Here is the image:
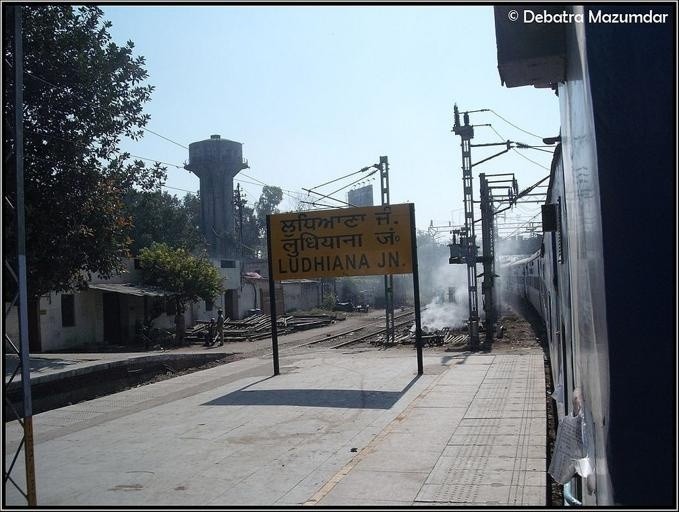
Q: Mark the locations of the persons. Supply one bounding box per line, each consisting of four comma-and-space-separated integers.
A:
214, 309, 224, 347
174, 308, 186, 348
203, 318, 217, 346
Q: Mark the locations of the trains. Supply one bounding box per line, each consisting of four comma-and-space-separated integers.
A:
494, 2, 677, 509
